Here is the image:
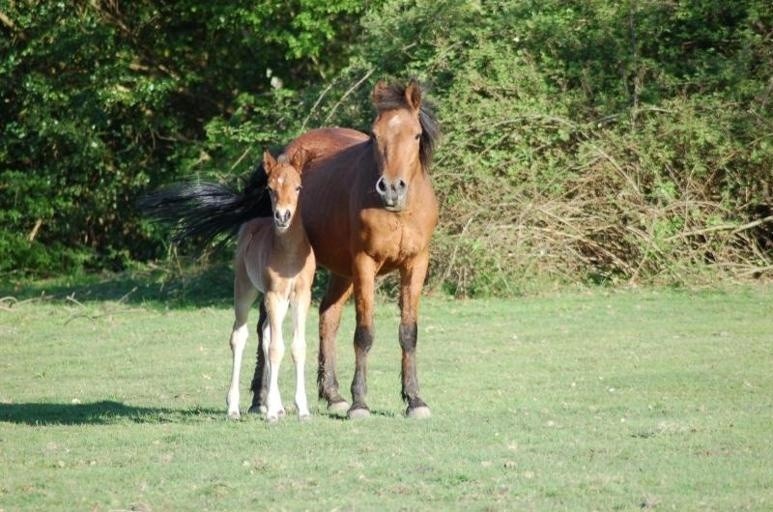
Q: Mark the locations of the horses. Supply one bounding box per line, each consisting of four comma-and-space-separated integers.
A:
225, 147, 316, 422
139, 76, 446, 422
